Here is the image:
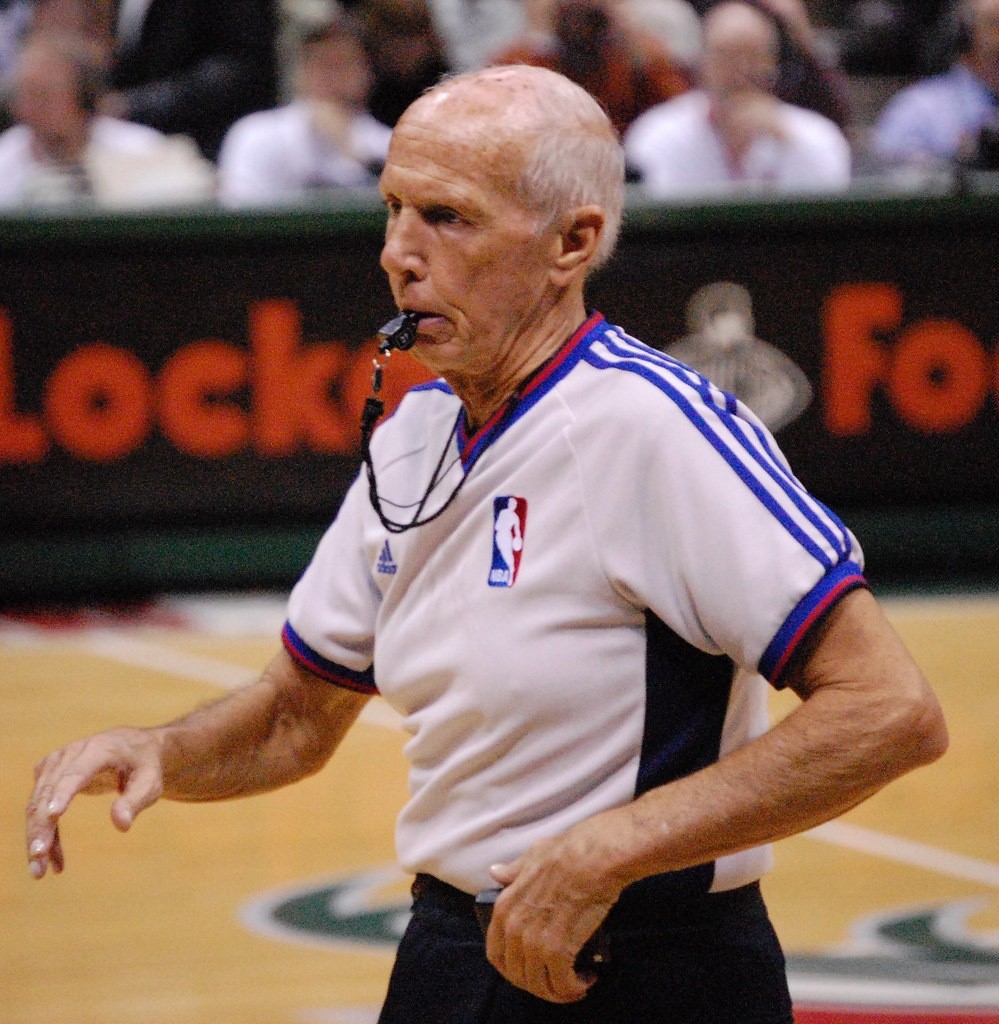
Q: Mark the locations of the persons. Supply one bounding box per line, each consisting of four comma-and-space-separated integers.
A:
0, 1, 999, 207
23, 62, 950, 1024
